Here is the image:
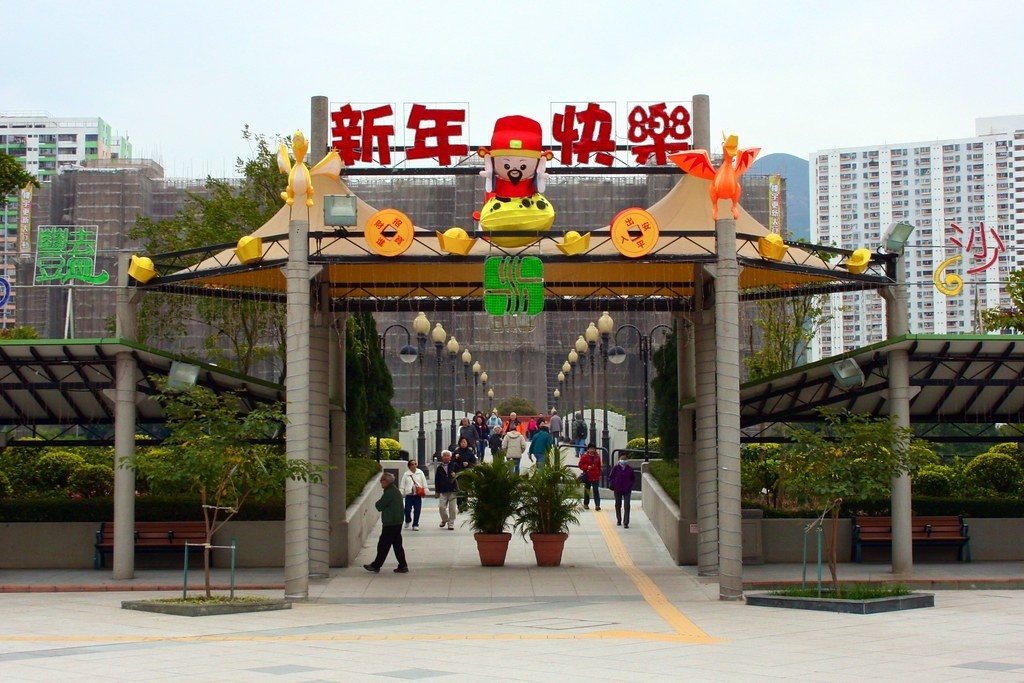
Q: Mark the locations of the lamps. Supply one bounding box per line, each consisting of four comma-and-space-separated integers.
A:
166, 361, 203, 393
879, 221, 917, 255
832, 358, 867, 387
322, 193, 362, 227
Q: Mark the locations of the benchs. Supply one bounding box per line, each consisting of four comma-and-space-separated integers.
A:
94, 521, 217, 569
848, 513, 974, 564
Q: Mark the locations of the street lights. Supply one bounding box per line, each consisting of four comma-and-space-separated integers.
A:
608, 323, 675, 461
413, 311, 430, 479
598, 311, 614, 466
373, 324, 418, 464
431, 322, 447, 463
568, 348, 578, 445
553, 389, 561, 412
585, 321, 600, 451
557, 371, 564, 442
574, 335, 588, 421
479, 371, 488, 415
561, 361, 571, 443
461, 348, 473, 418
446, 335, 460, 452
472, 360, 482, 415
487, 387, 496, 413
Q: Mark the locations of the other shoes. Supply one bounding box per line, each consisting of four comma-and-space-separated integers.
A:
439, 521, 446, 527
393, 568, 407, 573
595, 506, 600, 511
624, 524, 628, 528
412, 526, 419, 531
364, 564, 377, 571
584, 505, 589, 508
448, 525, 454, 530
617, 521, 621, 525
404, 521, 409, 528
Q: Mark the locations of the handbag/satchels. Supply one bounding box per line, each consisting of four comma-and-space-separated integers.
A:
416, 484, 425, 497
609, 467, 616, 490
451, 477, 459, 491
578, 473, 587, 483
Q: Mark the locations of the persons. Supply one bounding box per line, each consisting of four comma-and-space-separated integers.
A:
579, 442, 602, 511
607, 453, 635, 528
501, 424, 526, 475
529, 426, 553, 473
452, 437, 476, 512
478, 115, 553, 205
473, 411, 549, 446
363, 472, 409, 574
489, 426, 506, 465
460, 418, 479, 453
573, 413, 587, 458
434, 450, 464, 530
549, 411, 563, 447
400, 459, 429, 531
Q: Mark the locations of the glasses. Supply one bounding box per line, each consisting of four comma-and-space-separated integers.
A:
412, 464, 417, 465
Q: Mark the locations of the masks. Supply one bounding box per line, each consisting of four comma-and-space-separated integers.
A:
619, 459, 628, 464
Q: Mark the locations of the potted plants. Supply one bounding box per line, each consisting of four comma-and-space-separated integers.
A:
448, 447, 532, 567
512, 443, 592, 567
483, 396, 551, 424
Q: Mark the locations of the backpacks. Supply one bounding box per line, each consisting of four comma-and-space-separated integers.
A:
577, 420, 585, 438
